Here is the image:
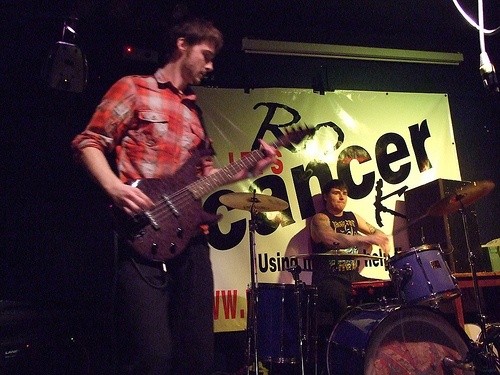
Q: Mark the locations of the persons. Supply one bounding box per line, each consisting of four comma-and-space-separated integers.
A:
69, 20, 277, 374
311, 179, 389, 315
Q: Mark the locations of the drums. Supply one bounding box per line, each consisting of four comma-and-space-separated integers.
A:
326, 302, 483, 375
245, 282, 319, 371
385, 243, 462, 309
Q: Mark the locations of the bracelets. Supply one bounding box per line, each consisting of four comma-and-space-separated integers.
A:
245, 166, 262, 177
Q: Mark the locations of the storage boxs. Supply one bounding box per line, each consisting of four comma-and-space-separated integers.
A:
405, 179, 483, 274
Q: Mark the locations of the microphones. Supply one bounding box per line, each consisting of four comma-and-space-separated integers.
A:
442, 356, 476, 371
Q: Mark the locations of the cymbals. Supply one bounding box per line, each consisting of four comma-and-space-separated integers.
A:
428, 181, 494, 216
290, 252, 386, 262
218, 192, 289, 212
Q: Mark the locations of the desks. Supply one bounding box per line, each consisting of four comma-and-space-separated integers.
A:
353, 272, 500, 329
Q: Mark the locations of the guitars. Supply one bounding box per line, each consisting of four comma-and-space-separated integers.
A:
112, 122, 318, 262
